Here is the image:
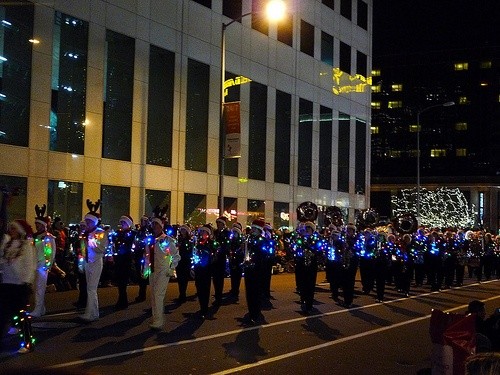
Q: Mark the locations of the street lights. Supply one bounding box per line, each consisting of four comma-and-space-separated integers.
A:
416, 100, 456, 215
216, 1, 288, 215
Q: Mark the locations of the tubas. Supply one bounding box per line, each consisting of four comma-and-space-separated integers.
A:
323, 206, 342, 226
296, 201, 319, 223
392, 212, 418, 234
358, 207, 380, 230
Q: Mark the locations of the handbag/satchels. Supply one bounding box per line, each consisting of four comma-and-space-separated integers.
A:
467, 300, 500, 355
429, 307, 477, 375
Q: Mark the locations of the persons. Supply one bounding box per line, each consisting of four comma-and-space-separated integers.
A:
0, 212, 500, 356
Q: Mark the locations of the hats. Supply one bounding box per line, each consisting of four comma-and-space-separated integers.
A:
232, 219, 273, 235
84, 199, 102, 225
142, 214, 148, 221
216, 216, 228, 226
200, 222, 212, 235
35, 204, 50, 232
151, 204, 169, 228
300, 221, 425, 244
180, 224, 191, 236
119, 215, 133, 228
9, 219, 33, 240
431, 228, 500, 241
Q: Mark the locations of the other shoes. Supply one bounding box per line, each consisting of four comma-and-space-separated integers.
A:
73, 302, 86, 308
149, 320, 164, 327
30, 304, 45, 317
292, 282, 464, 317
17, 346, 30, 353
78, 313, 99, 321
